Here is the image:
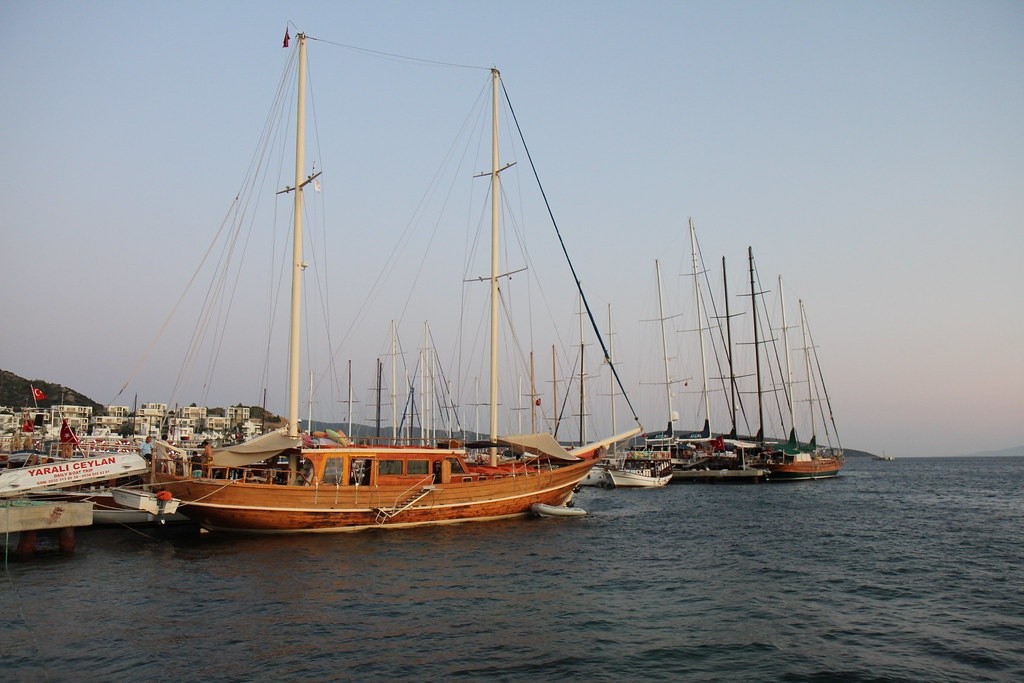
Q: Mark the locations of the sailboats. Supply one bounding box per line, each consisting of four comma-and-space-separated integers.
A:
0, 31, 845, 537
141, 33, 610, 536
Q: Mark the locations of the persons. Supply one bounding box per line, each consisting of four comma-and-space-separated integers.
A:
156, 434, 176, 474
188, 450, 203, 477
133, 436, 154, 461
201, 439, 214, 479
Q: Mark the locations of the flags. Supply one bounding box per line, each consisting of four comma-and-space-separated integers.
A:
21, 418, 34, 431
32, 386, 48, 399
60, 419, 79, 444
707, 436, 725, 451
641, 433, 648, 438
